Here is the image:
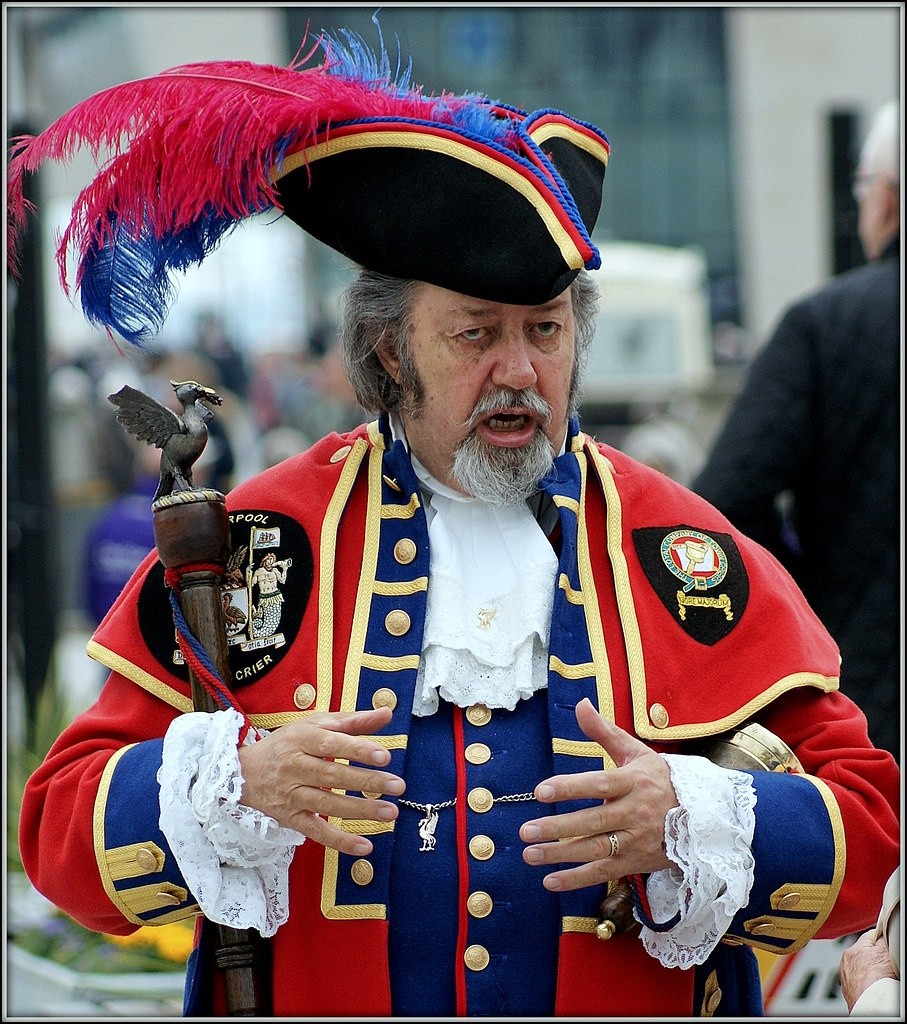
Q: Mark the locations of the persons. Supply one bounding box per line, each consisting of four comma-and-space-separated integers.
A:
37, 102, 903, 760
8, 13, 904, 1018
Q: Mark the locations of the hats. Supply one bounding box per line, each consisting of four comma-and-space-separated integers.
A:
7, 12, 610, 356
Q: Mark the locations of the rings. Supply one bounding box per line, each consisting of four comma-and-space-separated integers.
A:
605, 831, 619, 858
